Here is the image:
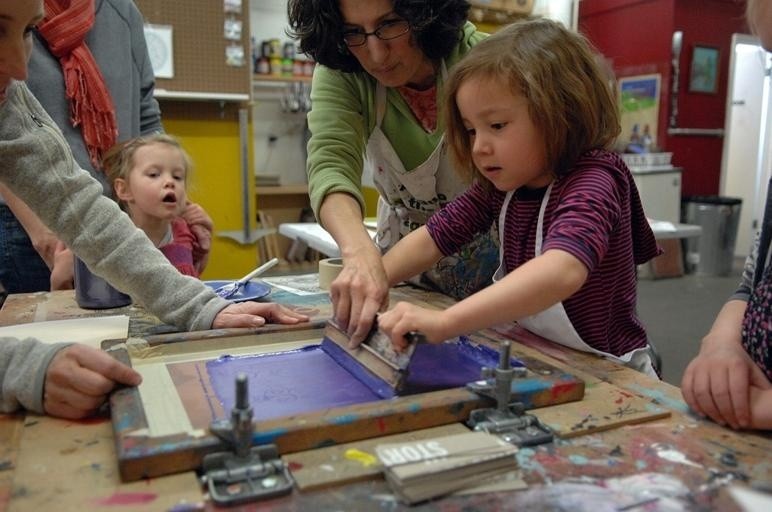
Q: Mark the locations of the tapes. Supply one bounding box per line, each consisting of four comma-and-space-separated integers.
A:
319, 257, 343, 292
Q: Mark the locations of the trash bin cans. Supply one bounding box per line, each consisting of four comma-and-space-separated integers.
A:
681, 194, 743, 276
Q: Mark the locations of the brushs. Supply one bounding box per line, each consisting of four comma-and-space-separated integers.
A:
216, 257, 279, 301
321, 304, 426, 399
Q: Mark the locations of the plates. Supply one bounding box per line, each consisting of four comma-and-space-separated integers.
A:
200, 281, 271, 303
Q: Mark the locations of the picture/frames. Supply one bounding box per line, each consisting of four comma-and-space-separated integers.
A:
615, 73, 662, 155
685, 41, 722, 97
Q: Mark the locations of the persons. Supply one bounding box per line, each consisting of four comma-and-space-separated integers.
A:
682, 2, 772, 434
378, 17, 663, 381
0, 0, 212, 292
0, 0, 310, 419
45, 132, 215, 291
286, 1, 497, 348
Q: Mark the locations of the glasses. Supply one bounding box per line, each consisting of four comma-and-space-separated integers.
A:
340, 16, 414, 46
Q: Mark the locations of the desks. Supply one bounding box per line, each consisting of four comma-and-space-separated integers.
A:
0, 274, 771, 512
